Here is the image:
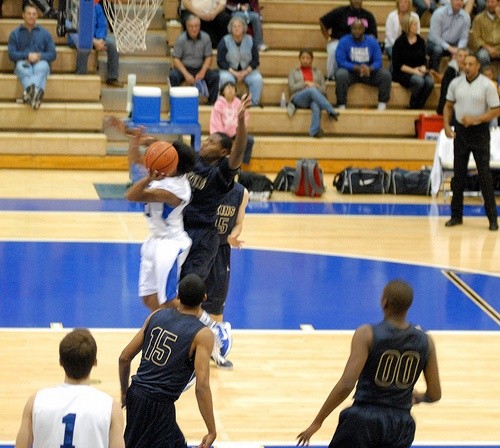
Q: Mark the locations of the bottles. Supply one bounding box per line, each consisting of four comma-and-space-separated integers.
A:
281, 92, 286, 108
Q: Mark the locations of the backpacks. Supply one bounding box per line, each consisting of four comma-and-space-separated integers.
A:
291, 159, 326, 198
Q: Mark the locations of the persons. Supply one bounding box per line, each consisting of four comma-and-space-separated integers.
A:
14, 328, 126, 448
334, 18, 392, 109
210, 81, 254, 164
8, 2, 56, 110
181, 0, 269, 51
169, 14, 220, 105
295, 279, 441, 448
119, 274, 216, 448
319, 0, 378, 80
443, 53, 500, 231
217, 16, 264, 109
32, 0, 57, 20
436, 48, 472, 126
481, 64, 500, 127
390, 14, 434, 109
412, 0, 500, 71
288, 48, 340, 138
384, 0, 420, 61
107, 92, 252, 392
68, 4, 124, 88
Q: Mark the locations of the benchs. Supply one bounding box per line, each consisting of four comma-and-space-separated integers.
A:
0, 0, 500, 175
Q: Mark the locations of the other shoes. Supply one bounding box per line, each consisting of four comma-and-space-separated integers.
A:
23, 87, 34, 103
332, 112, 340, 121
108, 78, 124, 88
56, 11, 66, 37
446, 218, 463, 226
489, 221, 498, 231
32, 91, 43, 109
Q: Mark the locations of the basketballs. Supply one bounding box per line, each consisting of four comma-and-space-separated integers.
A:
144, 140, 179, 177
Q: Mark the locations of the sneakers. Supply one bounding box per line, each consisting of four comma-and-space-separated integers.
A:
213, 321, 231, 357
211, 353, 233, 367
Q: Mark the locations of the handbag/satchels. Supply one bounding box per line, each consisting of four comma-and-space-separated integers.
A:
333, 167, 388, 194
388, 168, 431, 197
274, 167, 297, 191
239, 173, 274, 200
